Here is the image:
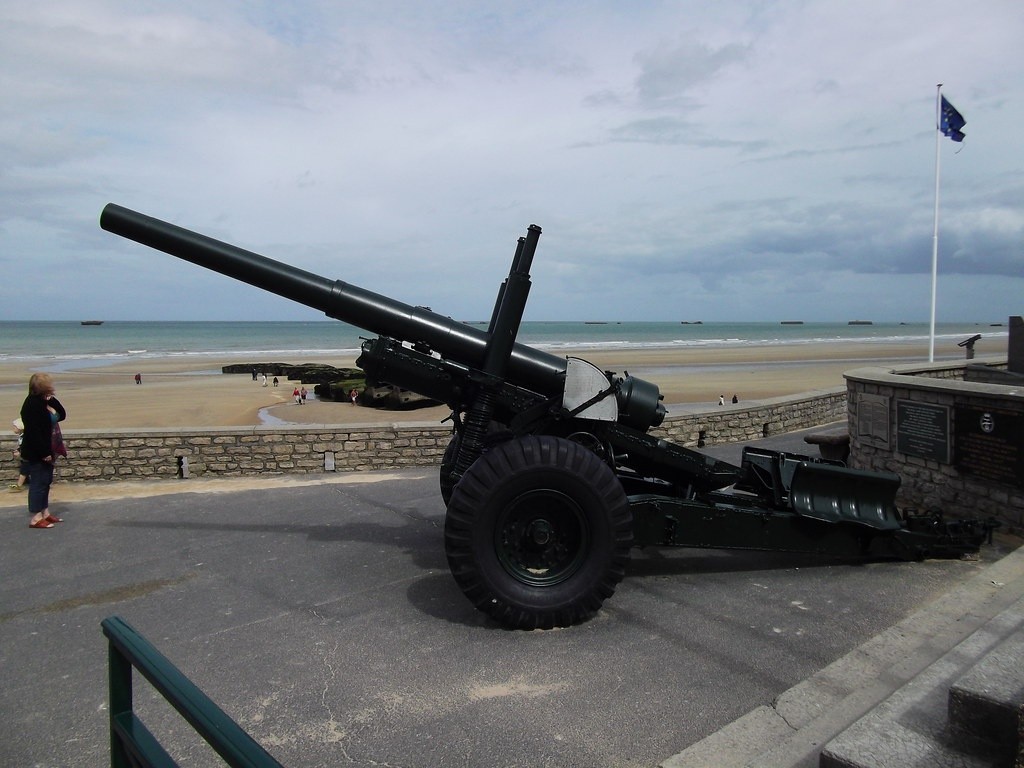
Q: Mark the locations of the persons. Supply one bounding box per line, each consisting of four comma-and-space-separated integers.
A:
351, 388, 358, 407
732, 394, 738, 403
19, 372, 68, 528
292, 387, 300, 405
301, 386, 308, 405
719, 394, 725, 406
7, 417, 33, 490
252, 368, 257, 381
137, 372, 142, 384
262, 372, 268, 387
273, 377, 278, 387
135, 375, 138, 384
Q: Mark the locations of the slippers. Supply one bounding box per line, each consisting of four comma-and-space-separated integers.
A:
46, 514, 64, 522
29, 518, 55, 528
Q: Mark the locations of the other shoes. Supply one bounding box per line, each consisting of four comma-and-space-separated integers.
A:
8, 484, 23, 493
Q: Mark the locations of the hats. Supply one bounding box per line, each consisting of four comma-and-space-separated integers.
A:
13, 417, 24, 430
